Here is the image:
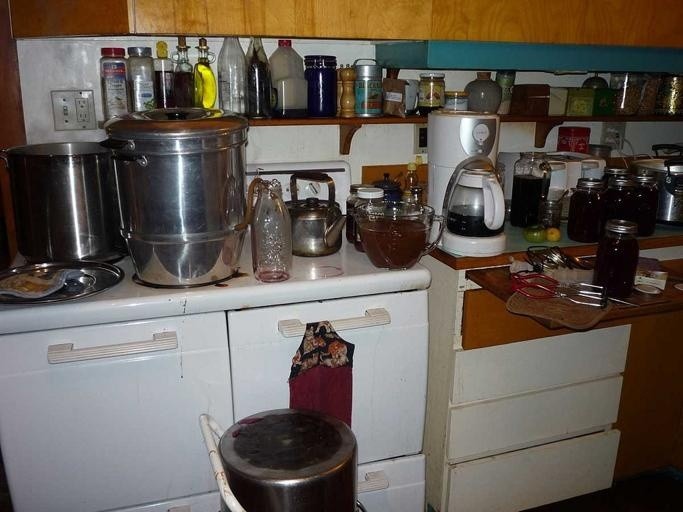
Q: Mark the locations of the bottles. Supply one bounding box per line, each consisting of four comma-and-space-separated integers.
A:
126, 46, 155, 113
252, 179, 291, 282
99, 46, 132, 125
353, 188, 385, 253
303, 54, 338, 116
417, 71, 446, 114
244, 37, 274, 120
216, 36, 249, 113
345, 184, 374, 243
401, 162, 417, 200
191, 37, 219, 107
508, 153, 551, 228
171, 36, 193, 106
153, 40, 175, 109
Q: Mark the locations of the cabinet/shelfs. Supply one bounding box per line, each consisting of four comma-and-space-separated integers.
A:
416, 253, 634, 512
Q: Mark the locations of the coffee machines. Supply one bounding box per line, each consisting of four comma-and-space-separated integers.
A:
427, 110, 507, 259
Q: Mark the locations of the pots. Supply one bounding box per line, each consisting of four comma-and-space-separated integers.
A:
218, 407, 366, 512
0, 141, 126, 269
629, 143, 683, 227
103, 106, 278, 291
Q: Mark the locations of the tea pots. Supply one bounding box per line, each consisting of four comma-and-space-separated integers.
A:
282, 169, 348, 258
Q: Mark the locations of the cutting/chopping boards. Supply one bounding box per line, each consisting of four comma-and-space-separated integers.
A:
505, 286, 612, 331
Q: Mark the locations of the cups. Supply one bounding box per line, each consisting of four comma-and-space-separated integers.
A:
352, 199, 445, 272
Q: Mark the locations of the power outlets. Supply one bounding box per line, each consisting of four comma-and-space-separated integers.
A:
50, 88, 97, 133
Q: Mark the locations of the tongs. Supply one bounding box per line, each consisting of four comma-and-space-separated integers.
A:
511, 272, 609, 311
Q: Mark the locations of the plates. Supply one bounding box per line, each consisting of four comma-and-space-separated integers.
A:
0, 260, 127, 304
